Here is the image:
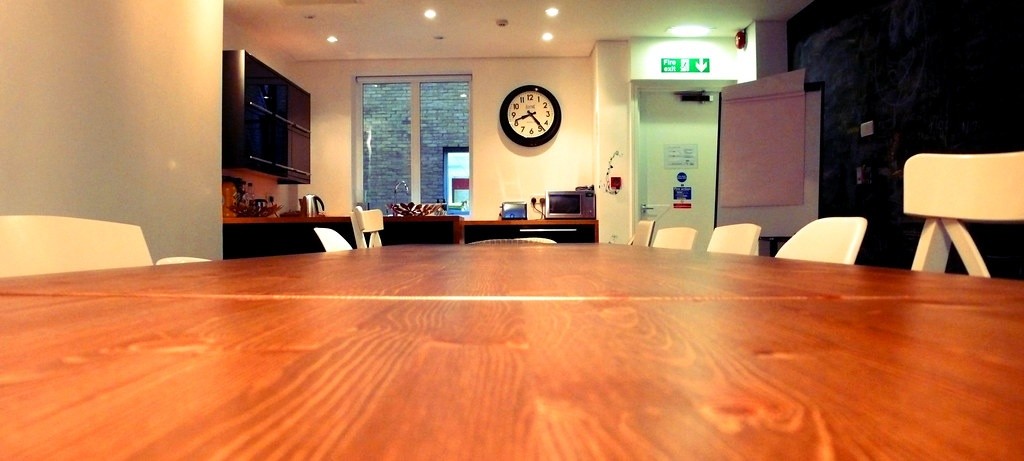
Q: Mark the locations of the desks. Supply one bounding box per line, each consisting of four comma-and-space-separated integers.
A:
0, 243, 1024, 461
463, 218, 599, 245
223, 216, 464, 259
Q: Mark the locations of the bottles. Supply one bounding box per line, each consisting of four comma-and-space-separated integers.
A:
244, 182, 255, 206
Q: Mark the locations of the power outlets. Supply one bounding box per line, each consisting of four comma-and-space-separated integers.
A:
531, 194, 545, 204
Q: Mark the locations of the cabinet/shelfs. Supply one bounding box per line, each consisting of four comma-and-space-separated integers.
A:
222, 50, 311, 185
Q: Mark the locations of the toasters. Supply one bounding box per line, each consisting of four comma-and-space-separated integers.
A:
499, 201, 527, 220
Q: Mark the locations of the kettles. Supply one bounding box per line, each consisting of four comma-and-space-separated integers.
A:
298, 194, 324, 217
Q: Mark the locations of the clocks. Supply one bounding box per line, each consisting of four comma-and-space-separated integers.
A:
499, 84, 562, 148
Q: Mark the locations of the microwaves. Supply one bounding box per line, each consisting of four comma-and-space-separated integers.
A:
545, 190, 595, 219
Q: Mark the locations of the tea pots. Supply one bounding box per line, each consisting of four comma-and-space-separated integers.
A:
251, 198, 267, 216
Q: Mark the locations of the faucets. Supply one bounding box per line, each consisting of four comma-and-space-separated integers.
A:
392, 180, 409, 216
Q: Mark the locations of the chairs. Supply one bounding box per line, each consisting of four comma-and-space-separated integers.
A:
707, 223, 761, 256
0, 214, 153, 277
653, 227, 699, 249
350, 205, 384, 249
902, 151, 1023, 279
627, 220, 655, 247
313, 228, 352, 252
774, 217, 868, 264
